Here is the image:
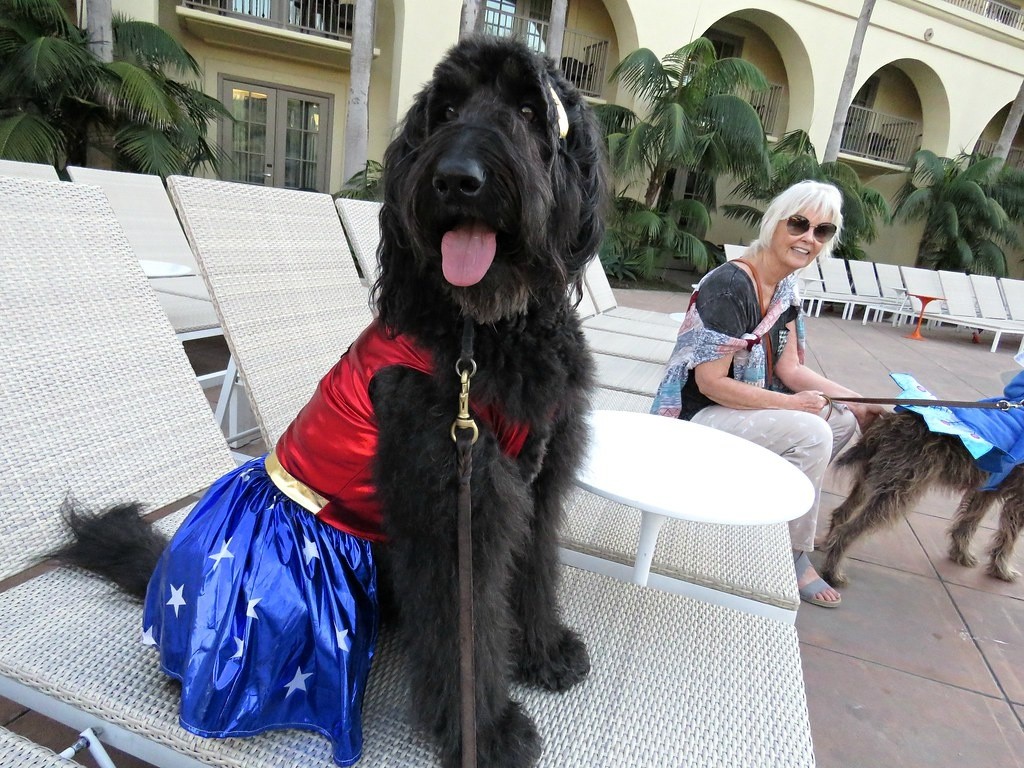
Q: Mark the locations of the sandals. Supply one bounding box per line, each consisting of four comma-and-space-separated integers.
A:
792, 548, 842, 609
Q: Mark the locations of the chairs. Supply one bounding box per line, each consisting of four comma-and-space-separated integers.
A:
0, 158, 1024, 768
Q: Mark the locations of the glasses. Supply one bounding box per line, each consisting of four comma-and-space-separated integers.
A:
784, 215, 838, 243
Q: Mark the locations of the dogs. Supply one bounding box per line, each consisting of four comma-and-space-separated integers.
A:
27, 34, 603, 767
819, 370, 1024, 590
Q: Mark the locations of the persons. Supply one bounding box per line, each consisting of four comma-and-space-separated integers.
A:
649, 181, 889, 609
